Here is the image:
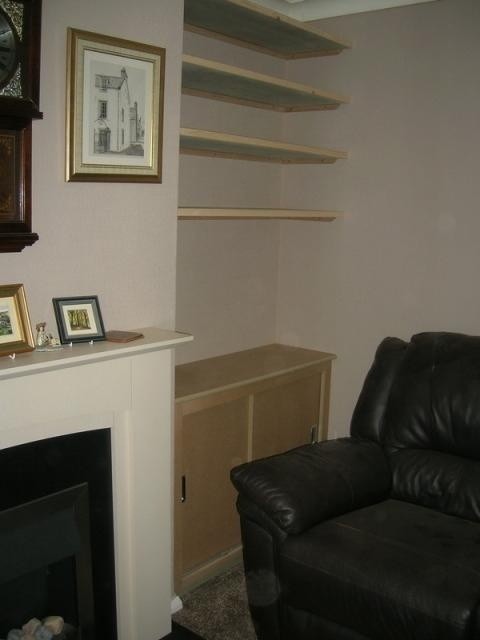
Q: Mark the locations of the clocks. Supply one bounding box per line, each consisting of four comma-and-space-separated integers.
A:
1, 0, 43, 254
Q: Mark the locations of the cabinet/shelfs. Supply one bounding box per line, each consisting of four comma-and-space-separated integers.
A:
173, 343, 337, 596
178, 0, 353, 221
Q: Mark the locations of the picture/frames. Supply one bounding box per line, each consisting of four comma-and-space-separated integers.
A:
65, 26, 166, 184
52, 295, 106, 344
0, 283, 35, 356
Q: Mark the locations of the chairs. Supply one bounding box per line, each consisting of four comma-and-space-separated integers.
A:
230, 332, 480, 640
